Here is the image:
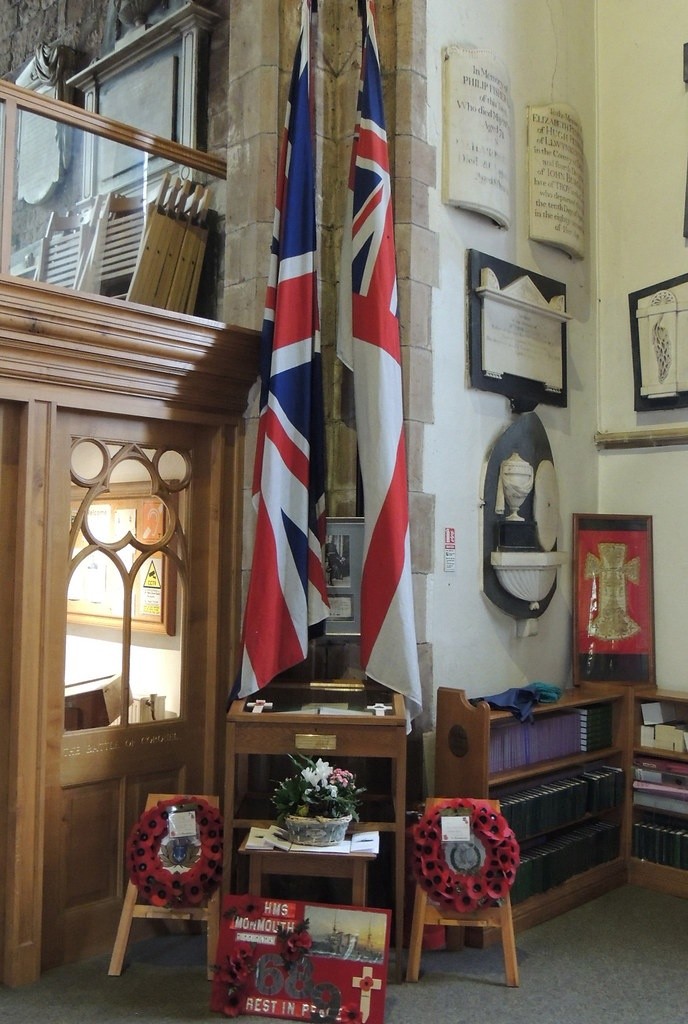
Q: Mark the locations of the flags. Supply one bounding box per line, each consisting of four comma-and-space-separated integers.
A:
336, 0, 424, 731
232, 0, 331, 703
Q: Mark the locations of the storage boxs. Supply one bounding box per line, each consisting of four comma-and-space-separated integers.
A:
641, 702, 676, 725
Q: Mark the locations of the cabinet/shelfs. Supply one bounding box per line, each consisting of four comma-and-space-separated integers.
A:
221, 681, 406, 983
435, 687, 688, 948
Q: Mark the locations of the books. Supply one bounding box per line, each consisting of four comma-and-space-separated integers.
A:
487, 705, 623, 907
632, 820, 688, 870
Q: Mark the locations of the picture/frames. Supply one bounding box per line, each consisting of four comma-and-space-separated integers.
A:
571, 512, 657, 690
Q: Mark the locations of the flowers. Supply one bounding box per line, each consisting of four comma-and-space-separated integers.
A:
408, 797, 520, 914
360, 976, 373, 991
269, 751, 368, 828
126, 796, 223, 907
210, 941, 256, 1017
340, 1003, 363, 1024
275, 918, 312, 974
222, 893, 265, 922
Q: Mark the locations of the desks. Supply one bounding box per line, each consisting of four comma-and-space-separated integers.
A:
237, 832, 376, 907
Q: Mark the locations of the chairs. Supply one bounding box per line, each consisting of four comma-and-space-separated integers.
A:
34, 172, 211, 315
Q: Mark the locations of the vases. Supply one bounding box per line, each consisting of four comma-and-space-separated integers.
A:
285, 815, 353, 846
